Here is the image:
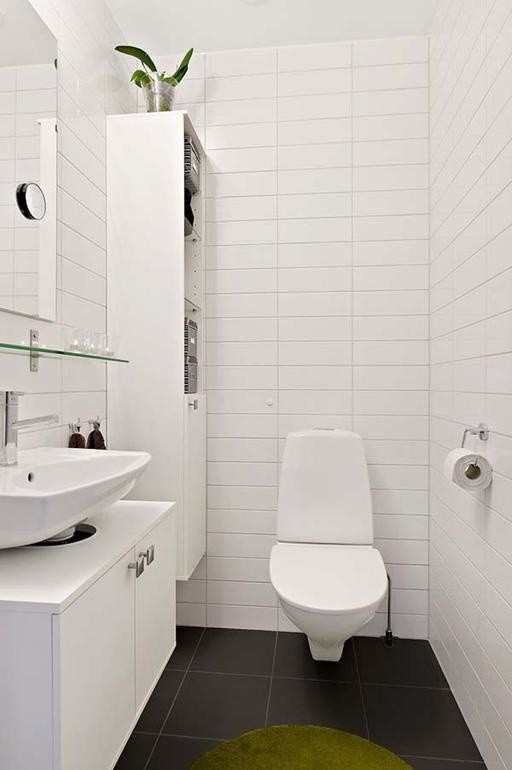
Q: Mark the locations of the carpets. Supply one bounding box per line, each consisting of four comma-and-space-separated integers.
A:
172, 721, 414, 769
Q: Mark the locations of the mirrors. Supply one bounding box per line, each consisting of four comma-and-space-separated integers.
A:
0, 0, 61, 330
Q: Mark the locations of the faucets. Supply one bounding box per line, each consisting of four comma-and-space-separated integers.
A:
0, 389, 60, 465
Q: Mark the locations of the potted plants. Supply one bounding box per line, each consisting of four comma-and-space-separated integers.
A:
113, 40, 194, 113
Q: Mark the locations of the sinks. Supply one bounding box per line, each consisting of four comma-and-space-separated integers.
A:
0, 446, 152, 548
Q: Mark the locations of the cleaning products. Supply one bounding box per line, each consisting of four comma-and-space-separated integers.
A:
68, 418, 85, 448
87, 418, 105, 449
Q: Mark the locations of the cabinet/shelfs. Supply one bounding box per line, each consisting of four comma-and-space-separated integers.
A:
0, 501, 176, 770
104, 113, 208, 582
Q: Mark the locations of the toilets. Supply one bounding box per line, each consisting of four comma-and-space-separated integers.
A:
271, 427, 388, 662
